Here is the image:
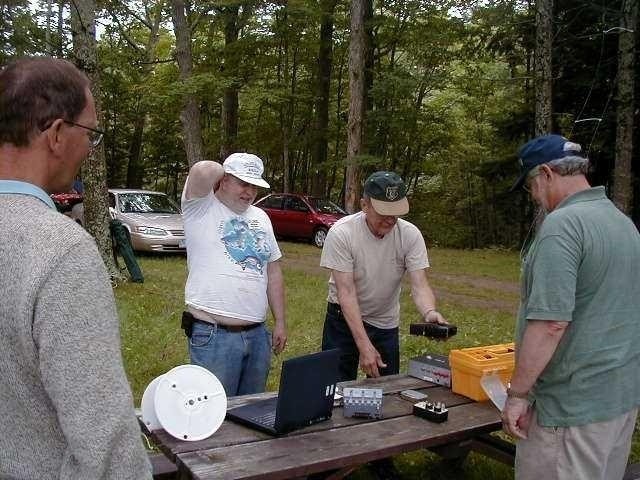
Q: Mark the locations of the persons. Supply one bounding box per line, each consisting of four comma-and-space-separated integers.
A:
499, 135, 640, 480
179, 152, 287, 399
0, 54, 156, 479
318, 170, 449, 479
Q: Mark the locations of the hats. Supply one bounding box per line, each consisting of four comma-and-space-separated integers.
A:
223, 153, 270, 189
508, 134, 580, 193
365, 171, 410, 216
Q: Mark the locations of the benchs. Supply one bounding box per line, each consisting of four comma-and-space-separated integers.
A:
147, 450, 180, 479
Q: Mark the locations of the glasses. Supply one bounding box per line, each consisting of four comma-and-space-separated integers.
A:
41, 120, 104, 148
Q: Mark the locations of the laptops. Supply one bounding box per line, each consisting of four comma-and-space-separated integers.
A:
225, 347, 343, 436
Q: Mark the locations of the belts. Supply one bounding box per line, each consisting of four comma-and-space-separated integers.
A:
193, 317, 264, 333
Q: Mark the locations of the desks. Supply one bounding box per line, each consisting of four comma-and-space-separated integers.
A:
129, 374, 505, 480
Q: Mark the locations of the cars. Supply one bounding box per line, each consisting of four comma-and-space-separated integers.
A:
251, 193, 353, 249
71, 188, 187, 254
49, 187, 83, 211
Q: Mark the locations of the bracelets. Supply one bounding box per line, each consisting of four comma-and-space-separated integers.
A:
422, 309, 436, 320
505, 381, 530, 399
